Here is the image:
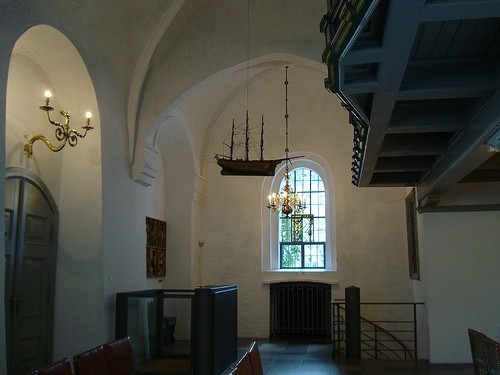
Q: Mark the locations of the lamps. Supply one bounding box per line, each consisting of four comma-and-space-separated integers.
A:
23, 90, 95, 158
268, 65, 304, 217
